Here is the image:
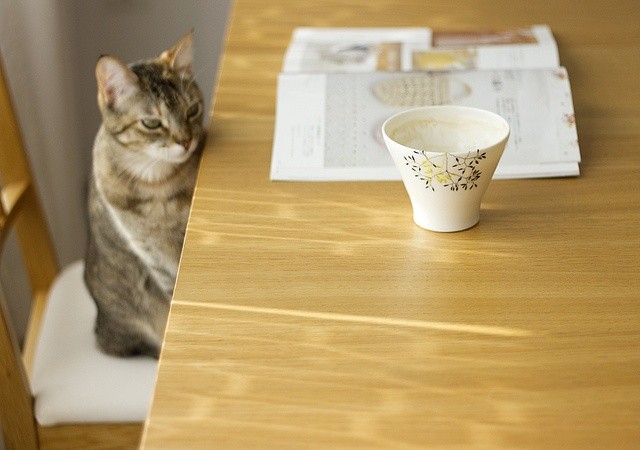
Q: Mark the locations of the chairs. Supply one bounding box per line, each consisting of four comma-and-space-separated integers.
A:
0, 55, 158, 450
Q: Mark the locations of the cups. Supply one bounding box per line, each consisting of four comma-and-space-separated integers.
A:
381, 105, 511, 232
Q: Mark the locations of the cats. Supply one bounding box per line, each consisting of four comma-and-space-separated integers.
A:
83, 27, 205, 360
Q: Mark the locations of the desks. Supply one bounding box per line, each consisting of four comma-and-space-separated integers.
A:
138, 1, 640, 448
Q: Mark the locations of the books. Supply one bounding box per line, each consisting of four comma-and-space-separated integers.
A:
271, 24, 581, 182
